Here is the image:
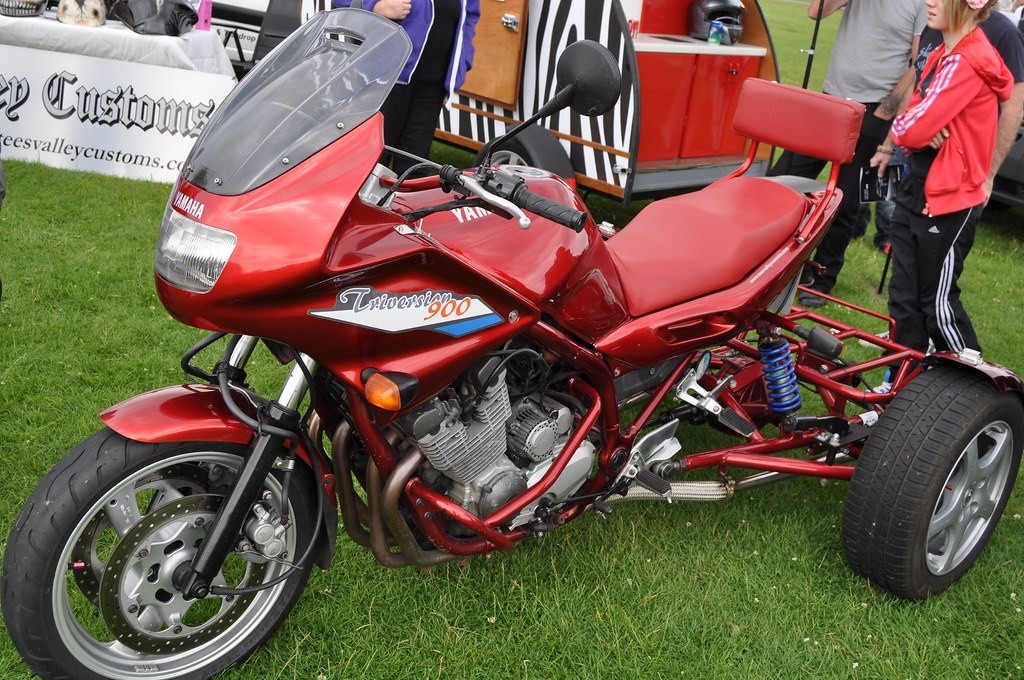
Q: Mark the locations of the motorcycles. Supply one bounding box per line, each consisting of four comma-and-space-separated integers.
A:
3, 9, 1023, 680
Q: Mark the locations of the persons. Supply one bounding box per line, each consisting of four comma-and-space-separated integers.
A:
328, 0, 483, 181
764, 0, 932, 308
865, 0, 1016, 399
858, 0, 1024, 360
996, 0, 1024, 35
851, 199, 898, 258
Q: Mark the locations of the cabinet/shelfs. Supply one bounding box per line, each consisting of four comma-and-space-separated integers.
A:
634, 33, 768, 163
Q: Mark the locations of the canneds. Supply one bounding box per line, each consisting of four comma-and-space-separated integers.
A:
708, 21, 722, 46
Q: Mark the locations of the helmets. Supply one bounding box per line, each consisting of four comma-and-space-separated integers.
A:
689, 0, 745, 47
